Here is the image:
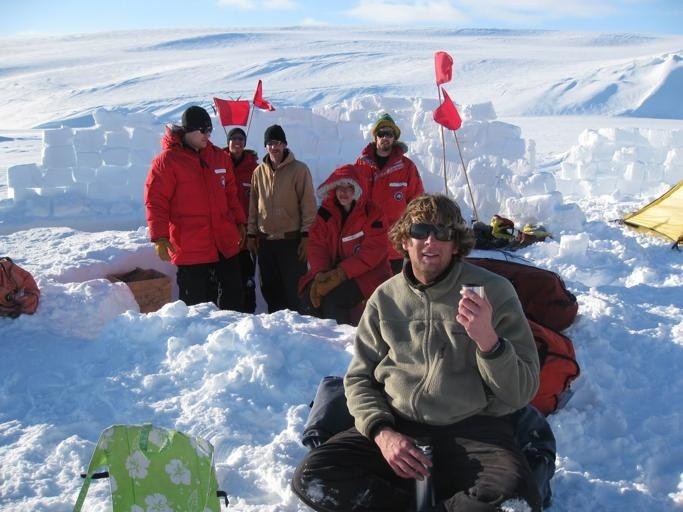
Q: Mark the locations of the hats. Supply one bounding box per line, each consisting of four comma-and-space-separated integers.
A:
181, 105, 211, 133
226, 128, 247, 145
263, 124, 287, 147
372, 113, 401, 140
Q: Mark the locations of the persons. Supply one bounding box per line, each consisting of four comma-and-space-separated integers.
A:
219, 129, 259, 316
288, 194, 555, 511
291, 164, 394, 327
355, 113, 427, 276
245, 124, 317, 316
142, 104, 248, 315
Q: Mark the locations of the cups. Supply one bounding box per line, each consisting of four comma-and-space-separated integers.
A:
459, 281, 486, 306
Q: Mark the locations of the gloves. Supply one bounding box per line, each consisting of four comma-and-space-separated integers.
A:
298, 237, 309, 261
309, 272, 321, 309
246, 236, 260, 253
237, 223, 248, 247
153, 237, 175, 261
317, 266, 345, 295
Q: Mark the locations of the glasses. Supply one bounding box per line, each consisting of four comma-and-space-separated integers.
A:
183, 127, 214, 134
404, 223, 456, 242
376, 130, 394, 139
229, 136, 243, 141
265, 140, 283, 145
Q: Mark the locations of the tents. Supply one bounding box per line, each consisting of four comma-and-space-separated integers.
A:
608, 179, 683, 249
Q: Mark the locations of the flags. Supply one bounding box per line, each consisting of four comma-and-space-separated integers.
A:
433, 50, 453, 87
212, 96, 250, 127
251, 80, 274, 112
432, 86, 462, 131
